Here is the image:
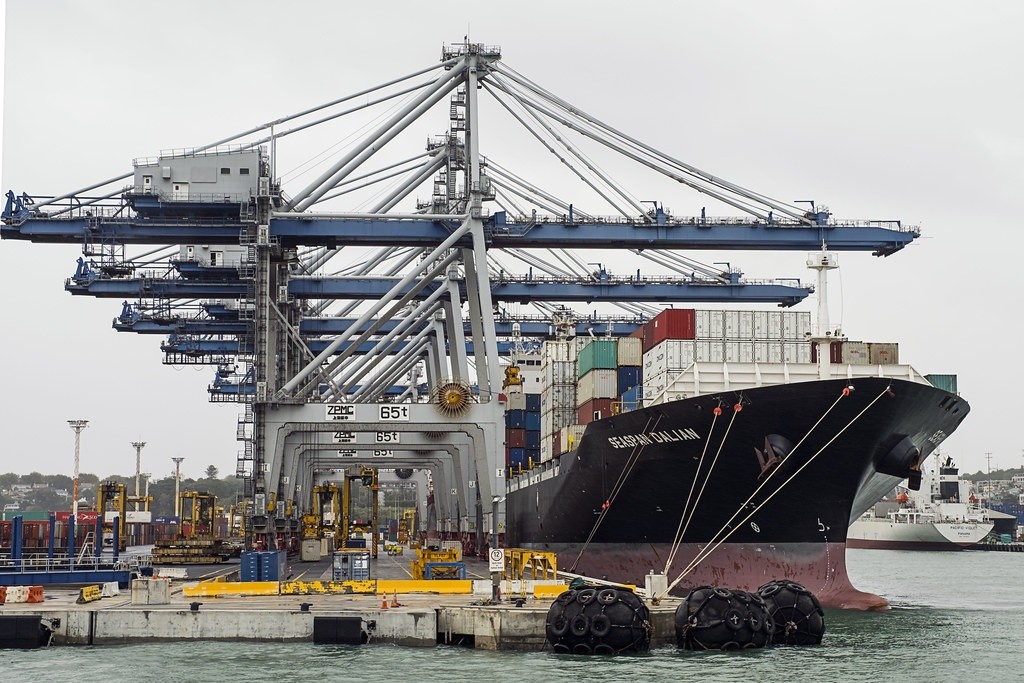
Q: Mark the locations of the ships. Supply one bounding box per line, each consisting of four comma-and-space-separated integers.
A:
502, 238, 973, 611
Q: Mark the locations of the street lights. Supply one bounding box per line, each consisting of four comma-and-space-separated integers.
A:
67, 420, 90, 538
171, 457, 185, 516
129, 441, 148, 511
141, 472, 152, 512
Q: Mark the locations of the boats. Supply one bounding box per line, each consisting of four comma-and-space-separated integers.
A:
846, 441, 995, 552
